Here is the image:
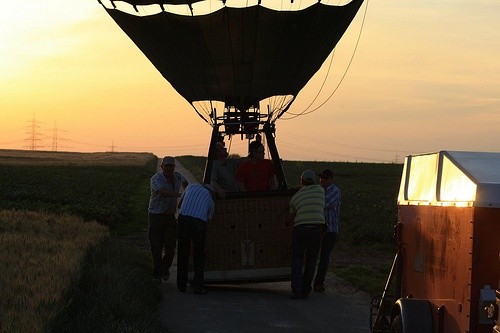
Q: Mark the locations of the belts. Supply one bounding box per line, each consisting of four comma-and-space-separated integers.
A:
295, 225, 322, 229
178, 217, 205, 225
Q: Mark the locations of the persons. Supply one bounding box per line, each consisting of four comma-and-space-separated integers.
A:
235, 142, 277, 193
177, 183, 216, 294
211, 142, 243, 196
288, 170, 326, 300
148, 156, 189, 285
310, 169, 342, 292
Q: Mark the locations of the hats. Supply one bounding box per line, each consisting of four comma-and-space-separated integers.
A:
301, 170, 315, 183
249, 141, 264, 154
318, 169, 333, 177
204, 184, 215, 200
162, 157, 176, 165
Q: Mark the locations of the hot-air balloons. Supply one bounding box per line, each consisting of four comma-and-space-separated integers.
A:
96, 0, 365, 288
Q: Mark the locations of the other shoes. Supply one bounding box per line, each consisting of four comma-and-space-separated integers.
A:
194, 289, 208, 295
299, 291, 308, 298
289, 292, 298, 299
314, 285, 325, 291
178, 286, 187, 293
161, 262, 171, 281
152, 275, 161, 283
